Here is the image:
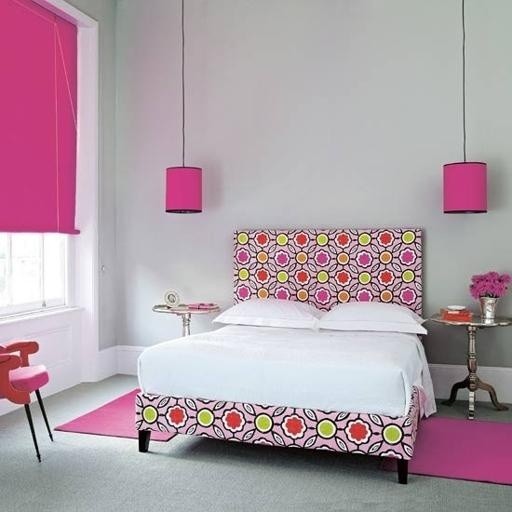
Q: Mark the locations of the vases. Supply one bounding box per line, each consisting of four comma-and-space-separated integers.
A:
480, 297, 502, 319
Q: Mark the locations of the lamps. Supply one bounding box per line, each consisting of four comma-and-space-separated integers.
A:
443, 1, 488, 213
165, 0, 202, 213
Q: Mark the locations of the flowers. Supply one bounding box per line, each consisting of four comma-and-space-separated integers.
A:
469, 270, 512, 300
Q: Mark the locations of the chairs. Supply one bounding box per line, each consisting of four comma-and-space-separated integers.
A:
0, 340, 53, 463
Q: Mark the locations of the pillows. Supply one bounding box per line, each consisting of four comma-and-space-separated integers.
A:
212, 298, 324, 330
320, 302, 429, 336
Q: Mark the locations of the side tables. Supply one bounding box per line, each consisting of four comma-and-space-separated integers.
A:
430, 314, 512, 419
152, 304, 220, 337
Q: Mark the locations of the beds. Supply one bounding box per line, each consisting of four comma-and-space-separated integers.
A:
136, 228, 438, 485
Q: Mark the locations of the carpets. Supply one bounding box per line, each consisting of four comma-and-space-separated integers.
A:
53, 387, 178, 442
376, 416, 512, 485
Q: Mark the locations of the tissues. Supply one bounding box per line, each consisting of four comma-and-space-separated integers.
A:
440, 305, 473, 322
